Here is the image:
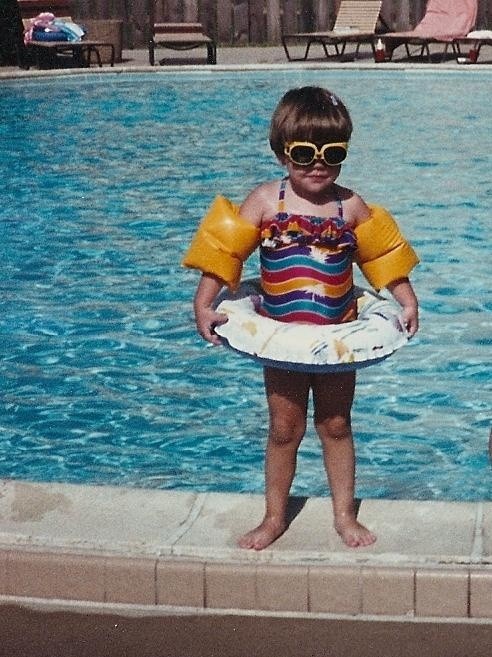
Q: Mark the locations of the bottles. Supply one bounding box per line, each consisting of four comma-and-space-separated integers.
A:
376, 39, 384, 62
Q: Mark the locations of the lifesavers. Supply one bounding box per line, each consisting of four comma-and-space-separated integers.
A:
351, 203, 420, 294
34, 24, 86, 42
212, 277, 411, 375
181, 194, 265, 294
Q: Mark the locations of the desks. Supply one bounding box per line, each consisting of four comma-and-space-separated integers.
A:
452, 38, 492, 64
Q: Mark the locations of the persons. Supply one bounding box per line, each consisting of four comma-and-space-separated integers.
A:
194, 82, 422, 554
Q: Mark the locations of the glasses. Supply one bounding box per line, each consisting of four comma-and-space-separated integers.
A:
284, 141, 348, 167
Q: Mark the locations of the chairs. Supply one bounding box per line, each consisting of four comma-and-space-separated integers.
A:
281, 1, 396, 63
375, 0, 478, 63
150, 22, 216, 66
21, 16, 116, 70
